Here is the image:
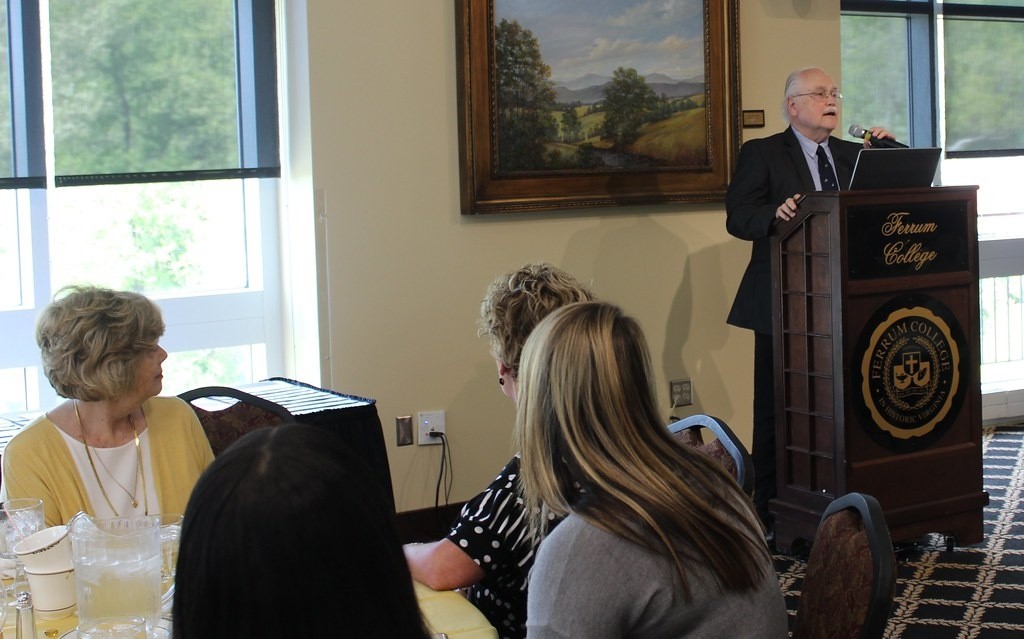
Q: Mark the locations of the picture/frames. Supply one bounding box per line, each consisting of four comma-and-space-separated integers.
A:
454, 0, 743, 214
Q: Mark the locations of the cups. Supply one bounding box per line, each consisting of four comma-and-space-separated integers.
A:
0, 498, 184, 639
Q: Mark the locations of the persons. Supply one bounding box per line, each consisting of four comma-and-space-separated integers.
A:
724, 68, 896, 556
403, 261, 789, 639
3, 284, 216, 529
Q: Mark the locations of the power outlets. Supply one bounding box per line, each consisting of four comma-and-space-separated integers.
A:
417, 410, 445, 445
670, 378, 693, 407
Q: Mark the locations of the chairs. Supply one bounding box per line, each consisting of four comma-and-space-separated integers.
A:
666, 414, 755, 501
790, 493, 896, 639
176, 386, 294, 460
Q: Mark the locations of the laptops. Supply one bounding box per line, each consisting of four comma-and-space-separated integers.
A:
849, 147, 943, 191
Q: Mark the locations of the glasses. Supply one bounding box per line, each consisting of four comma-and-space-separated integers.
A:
788, 86, 842, 103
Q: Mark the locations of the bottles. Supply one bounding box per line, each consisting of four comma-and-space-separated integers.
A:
15, 591, 39, 639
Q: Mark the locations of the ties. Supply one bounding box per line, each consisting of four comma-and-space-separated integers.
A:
816, 145, 839, 191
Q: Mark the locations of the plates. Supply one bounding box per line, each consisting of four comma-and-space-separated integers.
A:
60, 617, 174, 639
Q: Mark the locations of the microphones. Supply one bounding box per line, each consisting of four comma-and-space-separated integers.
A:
848, 125, 910, 149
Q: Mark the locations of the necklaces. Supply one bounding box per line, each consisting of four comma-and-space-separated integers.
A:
72, 399, 148, 516
